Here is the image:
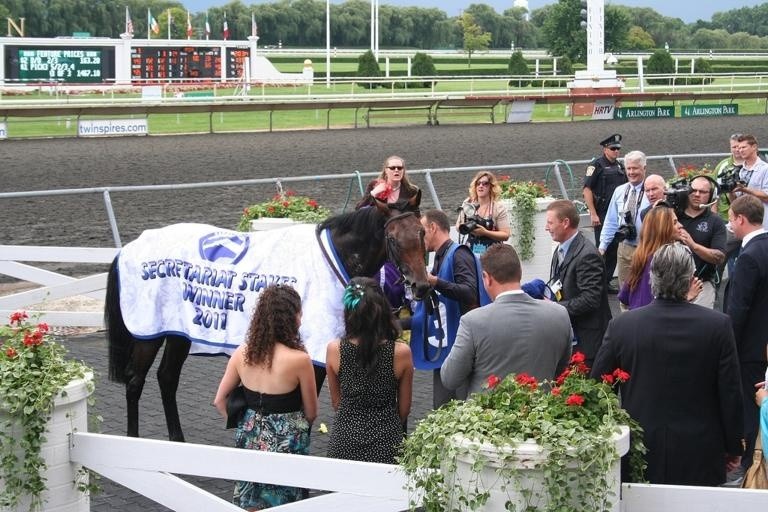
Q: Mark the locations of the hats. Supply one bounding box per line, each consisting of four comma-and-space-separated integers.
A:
599, 133, 621, 148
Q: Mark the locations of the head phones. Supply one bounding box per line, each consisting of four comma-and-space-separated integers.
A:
689, 173, 722, 201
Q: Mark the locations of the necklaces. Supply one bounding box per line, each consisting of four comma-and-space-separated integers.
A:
387, 183, 401, 192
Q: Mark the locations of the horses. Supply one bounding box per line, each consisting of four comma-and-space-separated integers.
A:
103, 187, 432, 443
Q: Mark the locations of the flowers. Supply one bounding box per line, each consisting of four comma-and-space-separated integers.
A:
233, 188, 333, 234
673, 159, 717, 195
397, 348, 652, 511
489, 173, 556, 265
0, 307, 110, 512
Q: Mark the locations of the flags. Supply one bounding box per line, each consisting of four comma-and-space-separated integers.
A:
168, 11, 181, 37
221, 15, 233, 39
204, 16, 216, 37
248, 15, 262, 36
186, 14, 196, 38
148, 11, 161, 35
127, 11, 135, 34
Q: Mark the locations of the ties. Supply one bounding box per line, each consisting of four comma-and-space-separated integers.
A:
557, 248, 567, 268
623, 186, 639, 230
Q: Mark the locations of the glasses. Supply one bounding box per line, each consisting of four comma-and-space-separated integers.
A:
605, 145, 622, 152
476, 179, 493, 187
386, 165, 405, 172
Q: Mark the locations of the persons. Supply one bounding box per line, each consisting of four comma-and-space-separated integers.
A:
325, 274, 414, 467
544, 199, 620, 396
440, 241, 573, 405
719, 192, 767, 488
213, 283, 319, 512
458, 173, 510, 254
589, 242, 744, 486
355, 152, 423, 312
750, 358, 766, 466
580, 127, 768, 318
395, 208, 486, 412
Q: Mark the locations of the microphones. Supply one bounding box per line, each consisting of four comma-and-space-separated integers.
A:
699, 196, 720, 209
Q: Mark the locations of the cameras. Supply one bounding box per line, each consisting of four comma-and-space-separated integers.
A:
459, 202, 494, 240
615, 211, 637, 242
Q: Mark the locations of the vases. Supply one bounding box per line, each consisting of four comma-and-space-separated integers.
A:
494, 198, 559, 289
250, 218, 294, 235
0, 370, 95, 512
440, 426, 630, 512
